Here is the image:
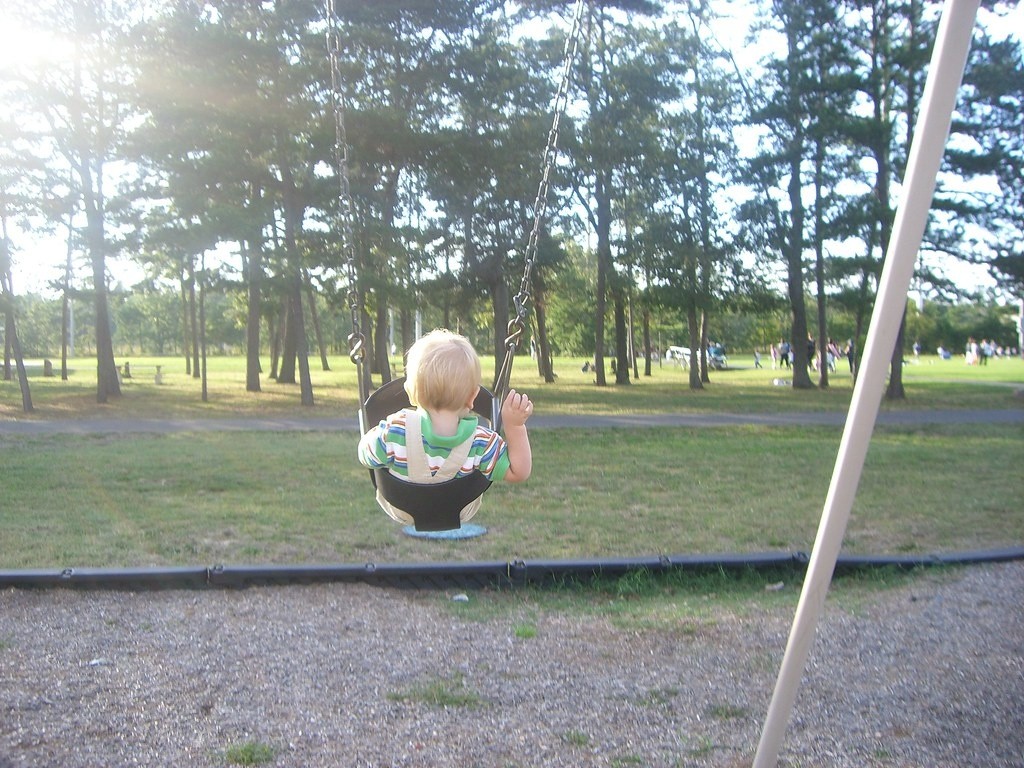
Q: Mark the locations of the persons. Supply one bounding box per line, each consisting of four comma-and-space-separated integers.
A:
771, 344, 777, 368
825, 336, 854, 372
913, 341, 921, 356
706, 338, 728, 369
780, 339, 791, 370
358, 329, 531, 527
937, 344, 948, 358
391, 342, 396, 355
965, 336, 1017, 366
790, 350, 794, 366
666, 348, 672, 360
753, 347, 763, 368
807, 332, 822, 371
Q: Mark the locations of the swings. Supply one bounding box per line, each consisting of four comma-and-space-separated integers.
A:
319, 1, 588, 538
